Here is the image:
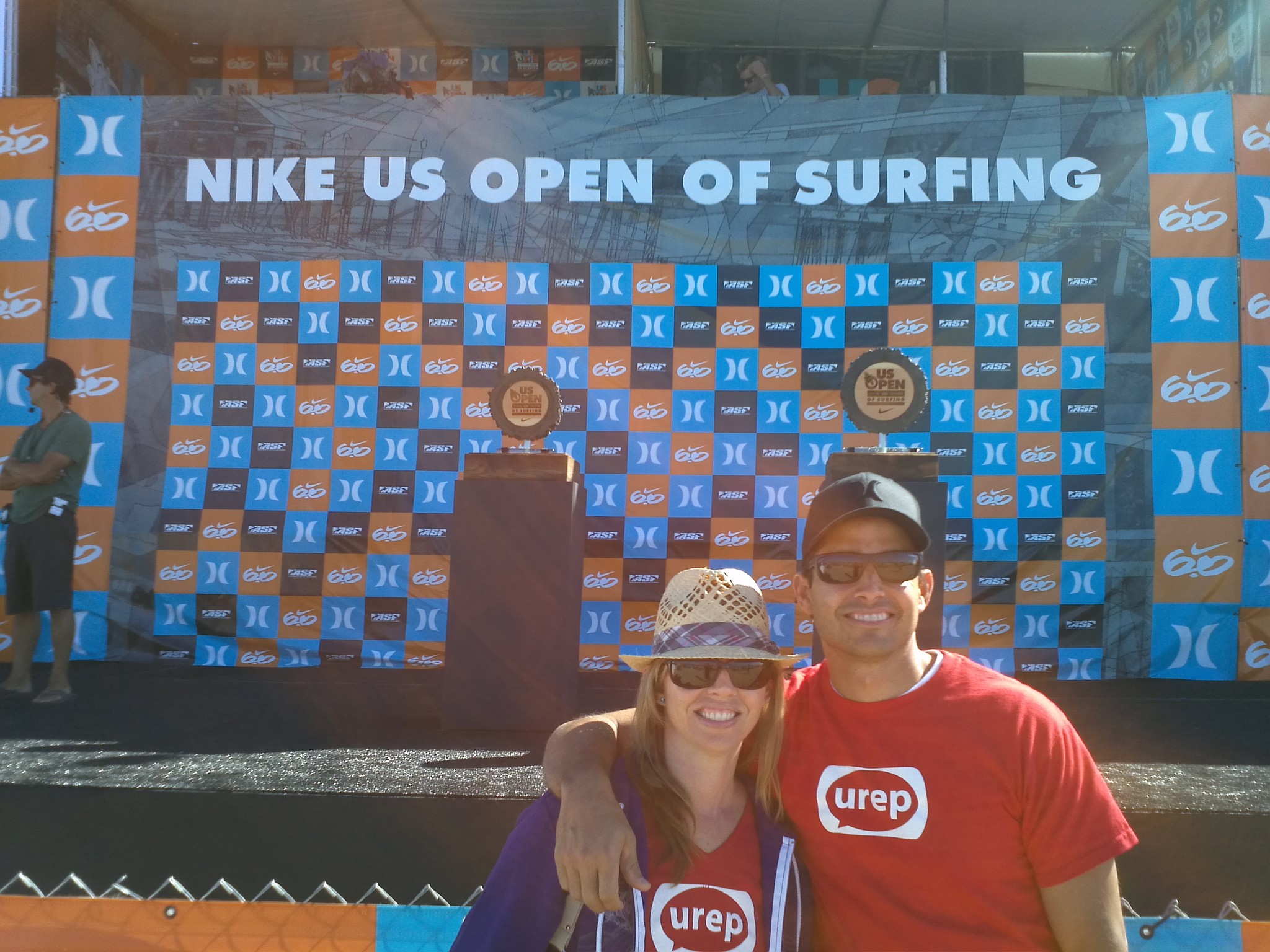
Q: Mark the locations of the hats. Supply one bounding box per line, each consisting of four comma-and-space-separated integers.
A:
801, 470, 931, 558
618, 566, 811, 675
17, 356, 77, 392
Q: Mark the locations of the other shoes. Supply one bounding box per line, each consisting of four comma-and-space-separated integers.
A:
29, 687, 74, 704
0, 685, 35, 699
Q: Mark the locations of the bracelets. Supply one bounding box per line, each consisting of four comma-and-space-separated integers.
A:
761, 73, 771, 81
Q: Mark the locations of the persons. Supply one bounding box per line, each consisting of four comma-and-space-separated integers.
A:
736, 53, 789, 96
544, 472, 1138, 952
450, 568, 817, 952
0, 357, 91, 705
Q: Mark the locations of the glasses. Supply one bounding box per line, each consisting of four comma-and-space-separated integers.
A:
28, 377, 45, 387
802, 548, 925, 585
662, 659, 775, 690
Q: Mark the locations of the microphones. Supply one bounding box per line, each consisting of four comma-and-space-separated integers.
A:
29, 406, 38, 413
